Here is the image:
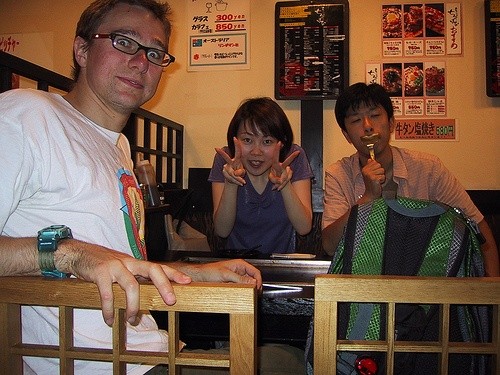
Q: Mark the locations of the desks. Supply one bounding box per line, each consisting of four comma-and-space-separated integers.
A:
164, 250, 332, 347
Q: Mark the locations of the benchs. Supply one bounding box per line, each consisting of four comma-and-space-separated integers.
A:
164, 189, 500, 257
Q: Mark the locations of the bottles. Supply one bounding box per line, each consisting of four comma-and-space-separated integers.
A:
132, 150, 160, 208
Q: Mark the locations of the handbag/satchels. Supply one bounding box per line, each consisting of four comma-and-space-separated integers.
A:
164, 192, 211, 251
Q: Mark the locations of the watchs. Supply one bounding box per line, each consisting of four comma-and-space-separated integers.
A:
36, 225, 74, 279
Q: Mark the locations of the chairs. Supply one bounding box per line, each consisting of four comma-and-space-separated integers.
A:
0, 275, 260, 375
315, 275, 500, 375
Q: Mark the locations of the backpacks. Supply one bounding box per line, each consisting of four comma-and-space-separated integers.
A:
306, 196, 497, 375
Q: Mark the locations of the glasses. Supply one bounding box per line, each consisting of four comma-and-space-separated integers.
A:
89, 33, 175, 67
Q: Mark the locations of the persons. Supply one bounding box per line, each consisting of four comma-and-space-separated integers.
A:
321, 82, 500, 278
208, 97, 315, 257
0, 0, 305, 375
